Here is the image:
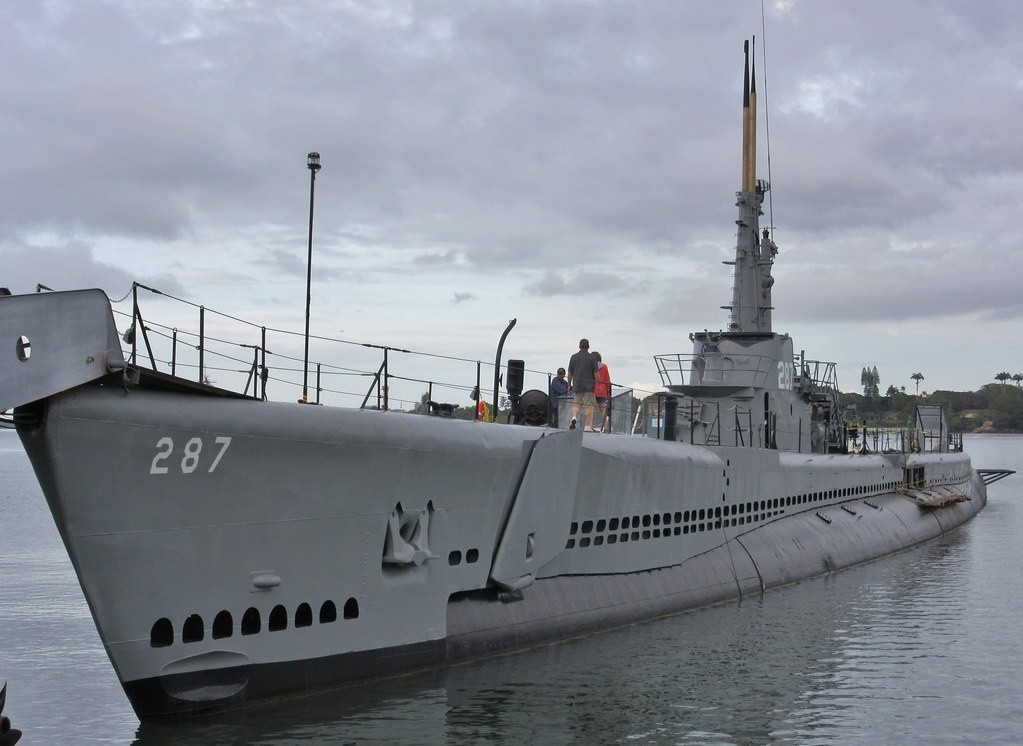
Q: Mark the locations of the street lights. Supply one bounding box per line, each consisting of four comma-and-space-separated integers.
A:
298, 150, 321, 405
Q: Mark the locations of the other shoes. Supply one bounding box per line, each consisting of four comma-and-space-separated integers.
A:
583, 425, 594, 432
570, 417, 577, 429
592, 427, 605, 433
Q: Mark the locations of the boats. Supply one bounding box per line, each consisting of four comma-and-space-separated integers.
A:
2, 34, 1019, 728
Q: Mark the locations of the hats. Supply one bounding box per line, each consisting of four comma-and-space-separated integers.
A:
579, 339, 589, 348
557, 368, 565, 374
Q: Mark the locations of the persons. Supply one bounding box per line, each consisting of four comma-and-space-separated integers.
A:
591, 352, 610, 433
550, 368, 568, 428
568, 339, 602, 432
